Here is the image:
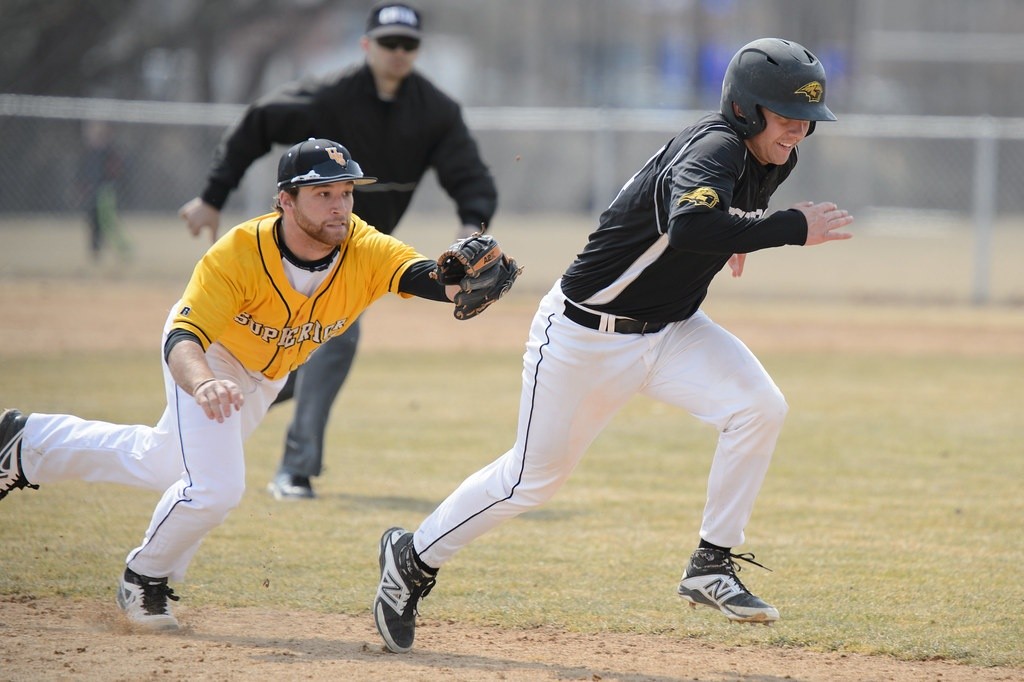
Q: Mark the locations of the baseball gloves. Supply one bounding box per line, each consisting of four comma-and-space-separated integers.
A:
430, 220, 524, 320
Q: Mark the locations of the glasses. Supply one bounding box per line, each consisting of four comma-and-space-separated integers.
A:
375, 33, 420, 52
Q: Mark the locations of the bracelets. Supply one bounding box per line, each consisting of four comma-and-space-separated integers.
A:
192, 377, 220, 397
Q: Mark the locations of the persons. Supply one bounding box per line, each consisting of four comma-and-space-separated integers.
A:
178, 4, 500, 502
373, 37, 856, 657
0, 133, 521, 632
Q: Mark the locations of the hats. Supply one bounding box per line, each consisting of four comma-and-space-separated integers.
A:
366, 4, 421, 38
277, 138, 378, 190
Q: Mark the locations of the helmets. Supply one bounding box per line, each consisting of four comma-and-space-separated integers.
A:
719, 37, 837, 140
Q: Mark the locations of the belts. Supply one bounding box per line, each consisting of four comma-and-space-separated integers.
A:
562, 299, 671, 335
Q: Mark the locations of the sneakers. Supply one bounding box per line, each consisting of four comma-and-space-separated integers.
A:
0, 409, 39, 499
677, 549, 780, 622
373, 526, 440, 654
114, 567, 180, 635
266, 470, 317, 500
